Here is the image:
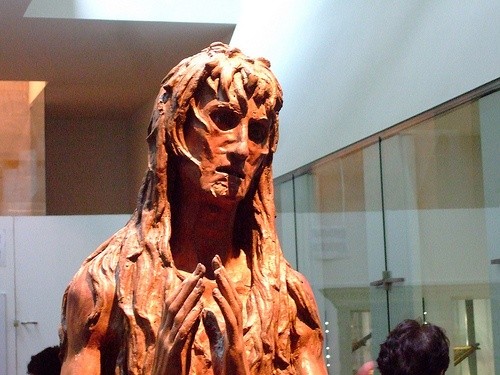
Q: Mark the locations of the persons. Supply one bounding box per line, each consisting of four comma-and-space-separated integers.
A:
61, 44, 332, 374
358, 318, 450, 374
